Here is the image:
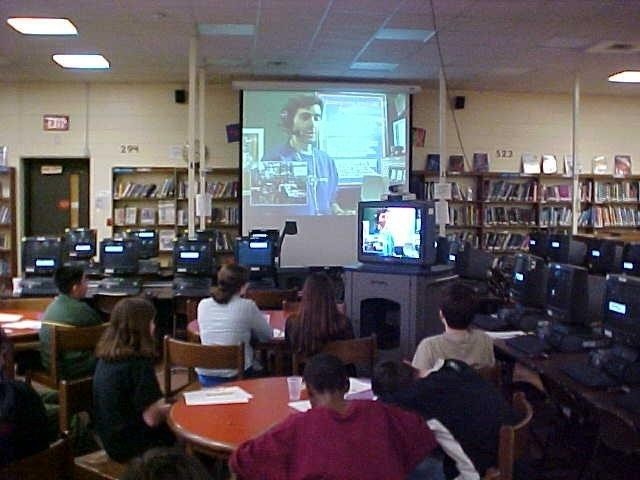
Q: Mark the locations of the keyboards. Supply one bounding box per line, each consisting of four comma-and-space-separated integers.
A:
472, 312, 512, 330
504, 335, 553, 354
92, 288, 140, 297
172, 288, 211, 299
21, 287, 61, 297
564, 362, 616, 388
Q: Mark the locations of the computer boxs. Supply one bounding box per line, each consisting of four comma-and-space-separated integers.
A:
497, 306, 542, 329
586, 347, 640, 375
544, 328, 611, 353
19, 277, 283, 289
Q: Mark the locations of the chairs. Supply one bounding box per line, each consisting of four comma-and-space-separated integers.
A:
472, 363, 534, 479
162, 288, 376, 396
2, 293, 128, 480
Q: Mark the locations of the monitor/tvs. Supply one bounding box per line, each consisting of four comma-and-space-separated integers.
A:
586, 240, 624, 275
67, 229, 97, 258
236, 236, 276, 273
546, 263, 606, 329
621, 244, 640, 276
99, 239, 139, 275
21, 237, 64, 277
129, 231, 159, 260
173, 241, 216, 277
601, 275, 639, 331
512, 253, 545, 309
548, 234, 588, 262
528, 232, 552, 253
358, 201, 436, 264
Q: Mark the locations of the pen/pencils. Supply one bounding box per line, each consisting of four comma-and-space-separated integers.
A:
206, 392, 233, 396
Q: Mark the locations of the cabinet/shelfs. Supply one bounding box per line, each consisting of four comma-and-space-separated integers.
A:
412, 168, 639, 257
0, 162, 19, 284
174, 165, 241, 270
108, 164, 176, 272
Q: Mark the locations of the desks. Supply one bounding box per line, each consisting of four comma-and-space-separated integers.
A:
461, 290, 640, 439
167, 376, 373, 453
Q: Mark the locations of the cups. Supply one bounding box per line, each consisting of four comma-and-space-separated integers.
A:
286, 377, 303, 400
12, 278, 23, 292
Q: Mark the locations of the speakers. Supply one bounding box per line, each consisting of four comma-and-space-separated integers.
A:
175, 90, 186, 103
455, 96, 465, 109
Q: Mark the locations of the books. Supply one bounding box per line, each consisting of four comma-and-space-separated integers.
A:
424, 154, 640, 249
114, 179, 239, 250
0, 201, 13, 251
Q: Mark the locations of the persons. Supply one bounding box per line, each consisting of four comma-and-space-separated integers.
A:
372, 358, 519, 480
286, 273, 354, 376
0, 266, 102, 463
229, 353, 439, 480
119, 447, 214, 480
91, 297, 177, 464
259, 96, 345, 215
195, 263, 274, 386
409, 280, 496, 380
370, 209, 395, 258
